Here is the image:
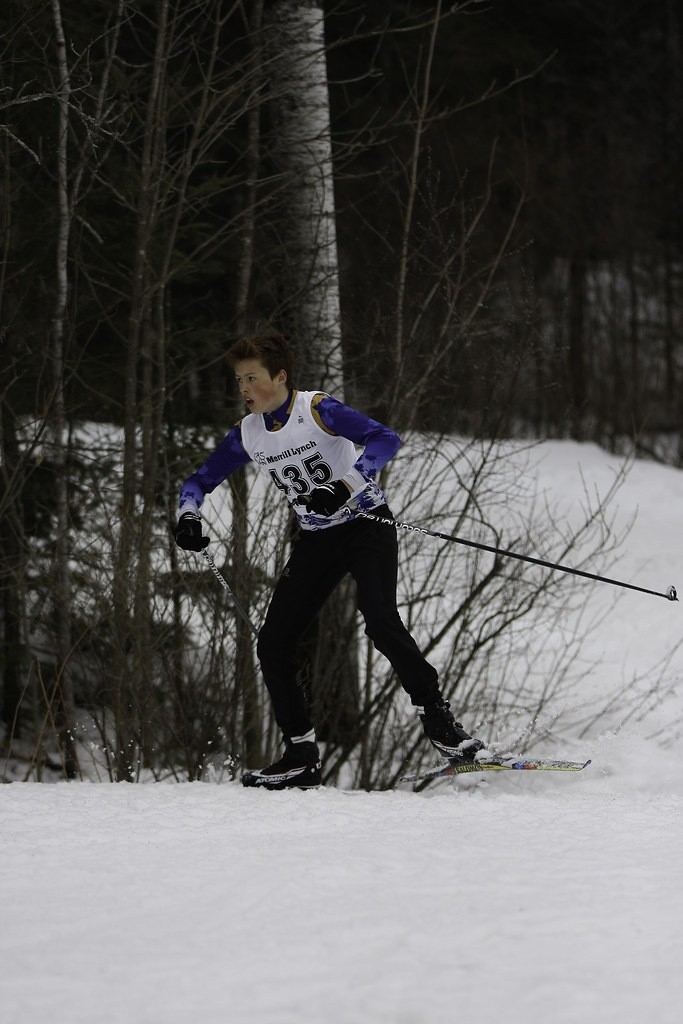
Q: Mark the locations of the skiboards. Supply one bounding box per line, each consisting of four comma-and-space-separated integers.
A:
396, 754, 592, 783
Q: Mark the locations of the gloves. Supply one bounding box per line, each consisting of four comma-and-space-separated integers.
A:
306, 479, 351, 517
174, 511, 210, 553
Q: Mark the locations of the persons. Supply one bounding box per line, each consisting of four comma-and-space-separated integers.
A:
175, 329, 487, 785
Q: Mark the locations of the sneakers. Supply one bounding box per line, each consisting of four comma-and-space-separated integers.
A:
242, 725, 323, 791
416, 690, 484, 757
